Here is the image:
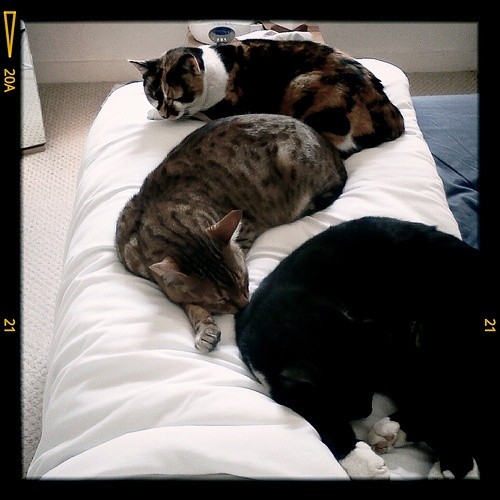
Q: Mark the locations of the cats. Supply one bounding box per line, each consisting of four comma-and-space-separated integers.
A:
128, 39, 405, 160
234, 216, 500, 479
115, 114, 348, 352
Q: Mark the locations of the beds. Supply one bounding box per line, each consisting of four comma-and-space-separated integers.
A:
26, 31, 478, 479
